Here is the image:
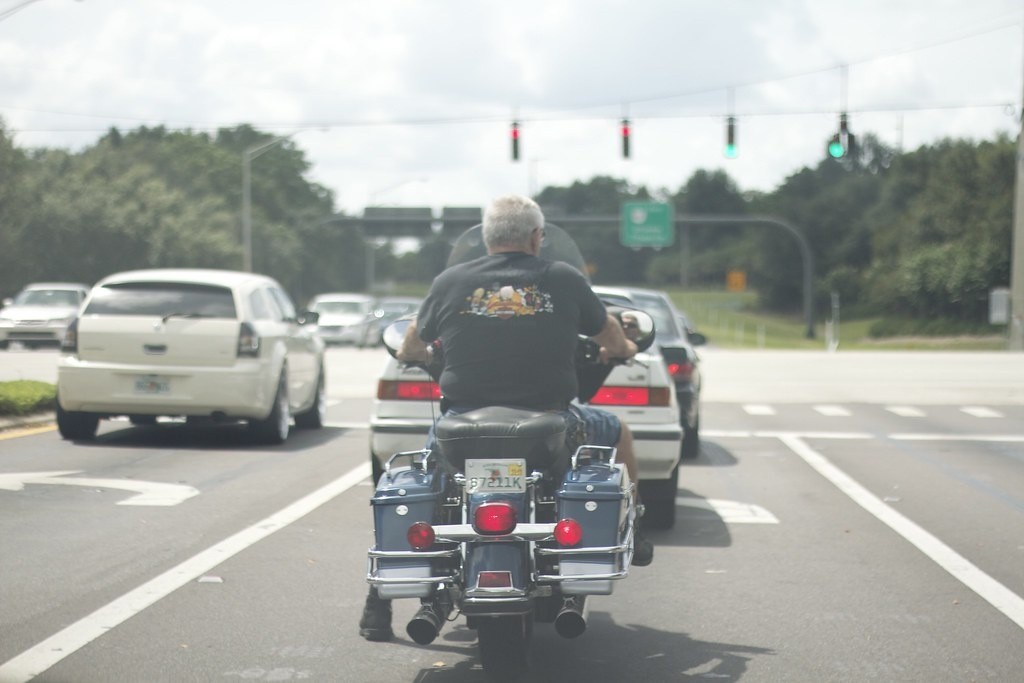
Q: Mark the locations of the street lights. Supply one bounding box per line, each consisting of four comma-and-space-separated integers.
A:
241, 126, 331, 274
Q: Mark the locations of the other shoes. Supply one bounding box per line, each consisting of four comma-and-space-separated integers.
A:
631, 531, 654, 566
359, 610, 392, 639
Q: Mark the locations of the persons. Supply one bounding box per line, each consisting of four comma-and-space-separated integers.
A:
361, 195, 655, 641
622, 314, 639, 340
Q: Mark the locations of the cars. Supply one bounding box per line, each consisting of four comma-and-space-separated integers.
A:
56, 269, 326, 442
308, 293, 426, 346
0, 282, 89, 349
369, 285, 707, 529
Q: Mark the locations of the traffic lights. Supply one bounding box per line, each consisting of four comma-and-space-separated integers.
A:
826, 129, 861, 174
722, 116, 741, 160
511, 124, 524, 166
620, 119, 631, 156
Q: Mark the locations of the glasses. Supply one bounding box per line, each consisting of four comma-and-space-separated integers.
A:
621, 321, 637, 329
533, 228, 547, 241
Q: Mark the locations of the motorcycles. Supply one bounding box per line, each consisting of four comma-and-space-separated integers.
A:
366, 299, 656, 677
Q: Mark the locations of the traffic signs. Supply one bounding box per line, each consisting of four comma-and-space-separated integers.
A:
619, 200, 676, 249
